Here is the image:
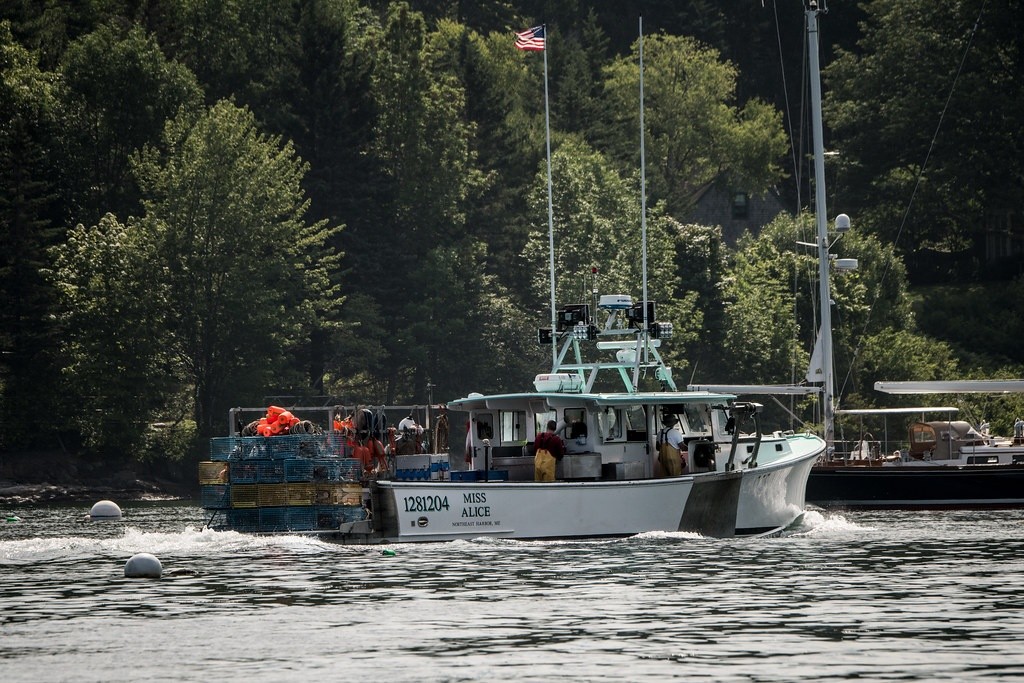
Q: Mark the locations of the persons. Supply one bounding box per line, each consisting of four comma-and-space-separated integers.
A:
656, 414, 688, 478
1014, 418, 1024, 437
532, 420, 564, 482
854, 433, 868, 451
980, 420, 989, 430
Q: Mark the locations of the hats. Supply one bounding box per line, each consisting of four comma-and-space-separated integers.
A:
662, 414, 678, 427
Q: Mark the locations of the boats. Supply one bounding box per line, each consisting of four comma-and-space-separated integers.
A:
197, 15, 828, 546
492, 0, 1024, 508
